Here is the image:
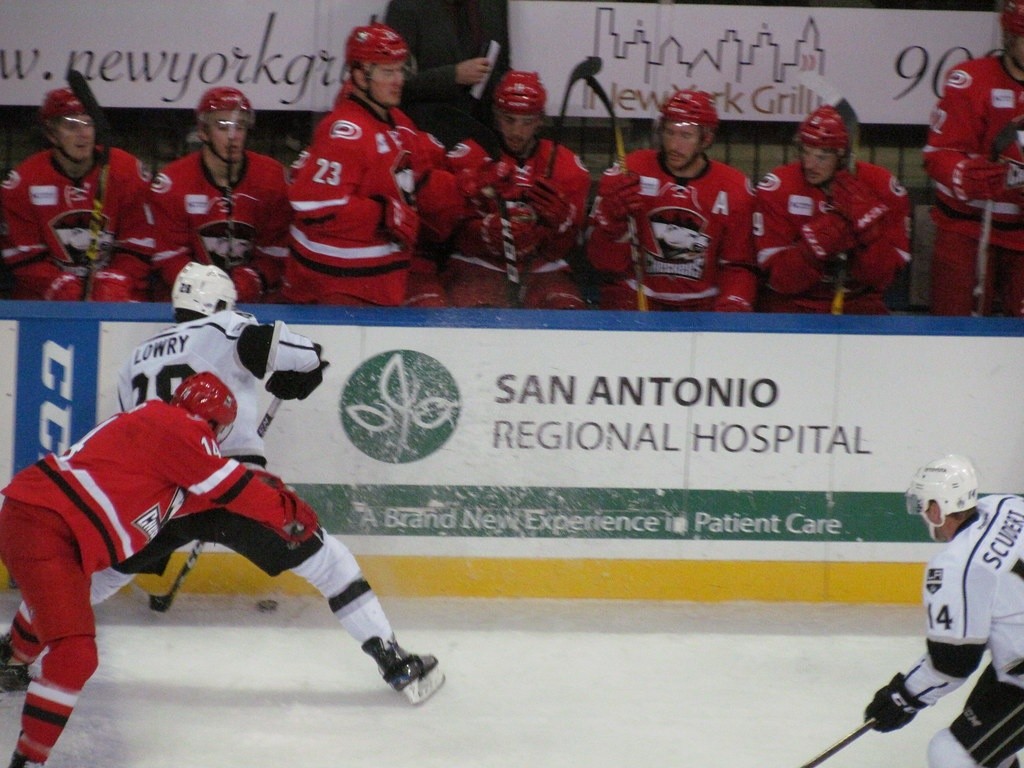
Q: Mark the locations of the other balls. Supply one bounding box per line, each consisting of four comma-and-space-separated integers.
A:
258, 599, 279, 612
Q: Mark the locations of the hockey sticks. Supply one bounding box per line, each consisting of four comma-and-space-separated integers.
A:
829, 98, 860, 313
125, 387, 279, 610
799, 717, 878, 768
972, 125, 1019, 315
540, 55, 653, 308
407, 36, 527, 302
60, 67, 112, 304
223, 54, 284, 277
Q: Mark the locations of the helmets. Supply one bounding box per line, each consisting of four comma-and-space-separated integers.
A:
171, 260, 238, 316
170, 371, 238, 444
346, 23, 409, 63
909, 455, 977, 515
799, 103, 849, 147
1000, 0, 1024, 35
197, 87, 249, 124
496, 71, 546, 114
663, 89, 717, 129
40, 87, 85, 118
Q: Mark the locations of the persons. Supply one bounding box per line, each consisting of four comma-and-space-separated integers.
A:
587, 92, 757, 313
920, 0, 1024, 318
282, 24, 591, 311
0, 88, 152, 303
753, 105, 913, 315
91, 263, 446, 704
0, 372, 318, 768
149, 86, 293, 303
864, 458, 1024, 768
385, 0, 511, 155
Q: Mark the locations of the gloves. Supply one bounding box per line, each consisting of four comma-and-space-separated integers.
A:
953, 153, 1010, 204
600, 173, 647, 222
234, 269, 263, 304
801, 210, 858, 257
864, 672, 929, 733
263, 489, 317, 544
524, 178, 572, 230
92, 268, 133, 301
380, 195, 427, 249
44, 274, 85, 300
265, 364, 326, 401
832, 172, 891, 243
486, 210, 546, 255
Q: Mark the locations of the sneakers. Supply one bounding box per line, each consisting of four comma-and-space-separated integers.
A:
361, 630, 444, 704
0, 634, 36, 697
